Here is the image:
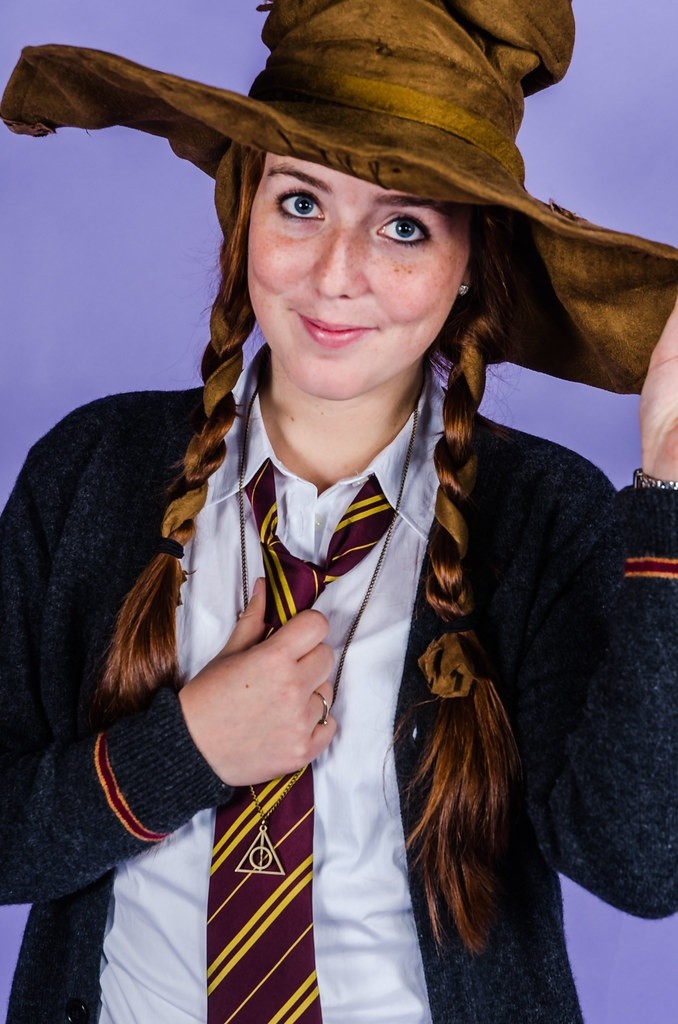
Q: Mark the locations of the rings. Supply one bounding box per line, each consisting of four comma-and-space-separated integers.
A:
312, 692, 328, 726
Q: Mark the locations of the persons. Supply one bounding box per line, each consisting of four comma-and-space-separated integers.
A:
0, 0, 678, 1024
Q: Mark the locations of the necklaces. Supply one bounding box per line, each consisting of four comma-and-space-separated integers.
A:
233, 365, 425, 876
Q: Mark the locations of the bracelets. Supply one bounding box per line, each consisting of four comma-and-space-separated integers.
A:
633, 468, 678, 490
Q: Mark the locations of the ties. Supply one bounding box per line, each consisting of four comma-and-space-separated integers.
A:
206, 458, 399, 1024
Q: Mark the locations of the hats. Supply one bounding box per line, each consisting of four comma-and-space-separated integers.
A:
0, 1, 678, 396
